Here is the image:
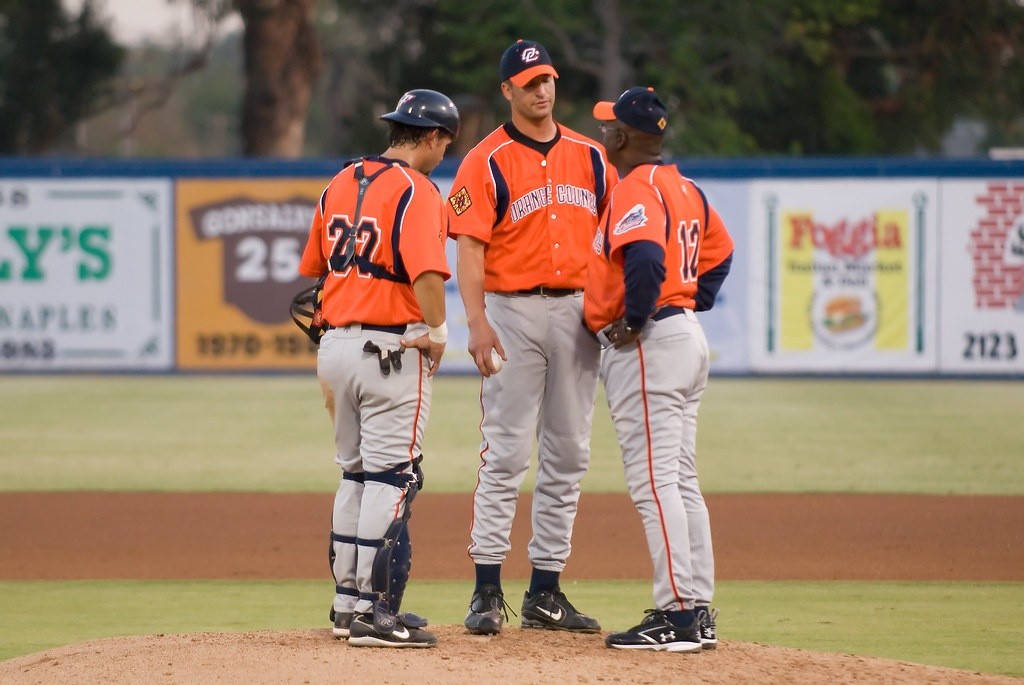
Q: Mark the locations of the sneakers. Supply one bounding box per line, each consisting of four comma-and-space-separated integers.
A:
520, 590, 601, 632
699, 608, 719, 649
348, 613, 438, 647
605, 608, 702, 653
465, 584, 517, 634
333, 612, 354, 636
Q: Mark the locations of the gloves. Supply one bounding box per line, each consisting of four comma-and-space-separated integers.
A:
363, 340, 406, 375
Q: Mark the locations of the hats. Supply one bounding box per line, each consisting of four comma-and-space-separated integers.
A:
498, 38, 559, 88
593, 87, 669, 135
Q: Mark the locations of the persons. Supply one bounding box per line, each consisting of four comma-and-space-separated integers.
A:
296, 87, 460, 648
584, 85, 734, 654
446, 39, 620, 635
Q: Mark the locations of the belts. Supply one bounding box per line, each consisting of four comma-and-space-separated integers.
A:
605, 304, 685, 342
328, 321, 407, 335
515, 286, 584, 297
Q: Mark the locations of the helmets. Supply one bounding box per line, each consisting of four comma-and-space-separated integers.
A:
379, 89, 459, 137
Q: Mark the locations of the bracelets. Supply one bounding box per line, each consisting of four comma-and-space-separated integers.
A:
427, 320, 448, 343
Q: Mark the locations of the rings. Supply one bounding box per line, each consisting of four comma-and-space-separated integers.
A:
614, 333, 618, 339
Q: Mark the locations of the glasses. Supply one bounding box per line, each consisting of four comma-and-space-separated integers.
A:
598, 123, 627, 135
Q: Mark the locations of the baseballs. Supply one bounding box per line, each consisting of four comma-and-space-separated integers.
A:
488, 353, 503, 375
